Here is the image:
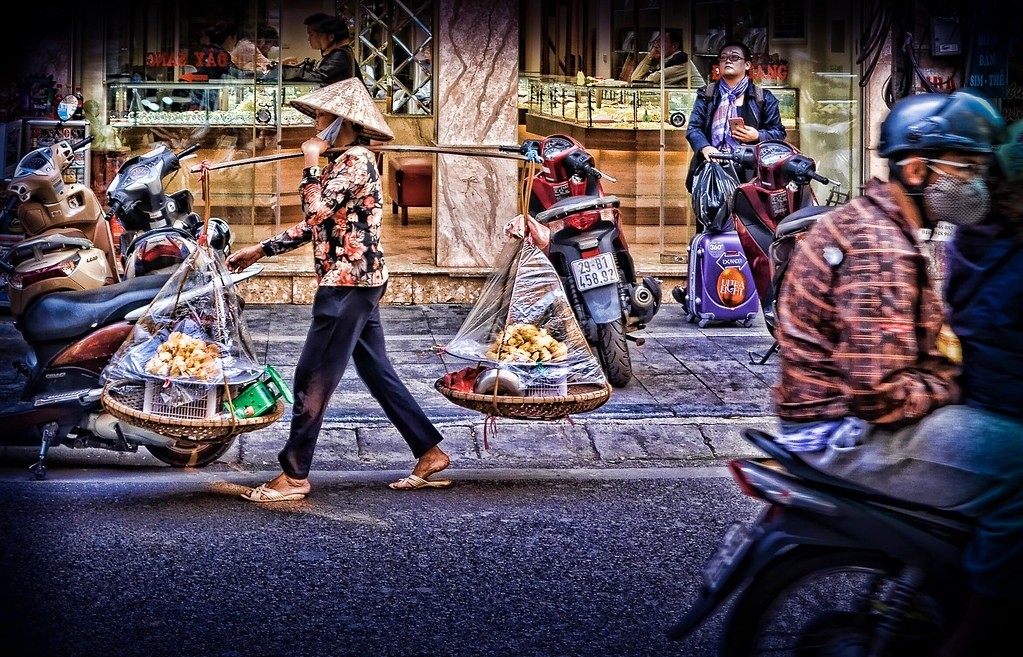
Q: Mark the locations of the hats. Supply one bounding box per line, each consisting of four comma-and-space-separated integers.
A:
290, 77, 394, 141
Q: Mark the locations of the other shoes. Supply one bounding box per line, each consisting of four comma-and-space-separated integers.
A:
672, 286, 689, 315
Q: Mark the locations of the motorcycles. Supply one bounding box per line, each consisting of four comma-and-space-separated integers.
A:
0, 262, 266, 480
0, 93, 118, 324
666, 426, 1023, 657
499, 135, 661, 389
707, 138, 842, 364
106, 140, 234, 275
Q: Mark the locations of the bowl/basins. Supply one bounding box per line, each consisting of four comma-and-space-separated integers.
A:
473, 368, 526, 397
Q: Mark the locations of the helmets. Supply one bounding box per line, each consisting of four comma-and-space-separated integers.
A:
877, 92, 1005, 158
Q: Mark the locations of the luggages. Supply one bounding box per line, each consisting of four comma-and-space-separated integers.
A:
685, 230, 759, 328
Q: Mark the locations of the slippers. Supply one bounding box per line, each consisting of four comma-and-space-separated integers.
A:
389, 474, 451, 490
241, 483, 305, 502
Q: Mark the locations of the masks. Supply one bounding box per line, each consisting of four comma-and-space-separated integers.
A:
923, 165, 991, 227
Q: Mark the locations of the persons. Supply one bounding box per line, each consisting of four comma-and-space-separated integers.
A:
303, 13, 370, 94
671, 43, 787, 315
776, 90, 1023, 657
630, 30, 706, 87
230, 27, 298, 72
224, 78, 452, 502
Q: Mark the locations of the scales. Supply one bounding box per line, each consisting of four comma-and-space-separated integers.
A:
224, 364, 295, 420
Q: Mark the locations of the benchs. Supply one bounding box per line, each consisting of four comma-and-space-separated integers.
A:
388, 157, 432, 226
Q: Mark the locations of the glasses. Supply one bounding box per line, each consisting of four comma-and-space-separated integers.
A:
718, 55, 744, 62
895, 156, 993, 178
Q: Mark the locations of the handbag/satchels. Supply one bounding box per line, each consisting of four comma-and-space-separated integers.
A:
692, 153, 740, 232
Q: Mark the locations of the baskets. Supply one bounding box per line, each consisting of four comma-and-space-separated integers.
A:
435, 365, 611, 422
102, 382, 283, 445
